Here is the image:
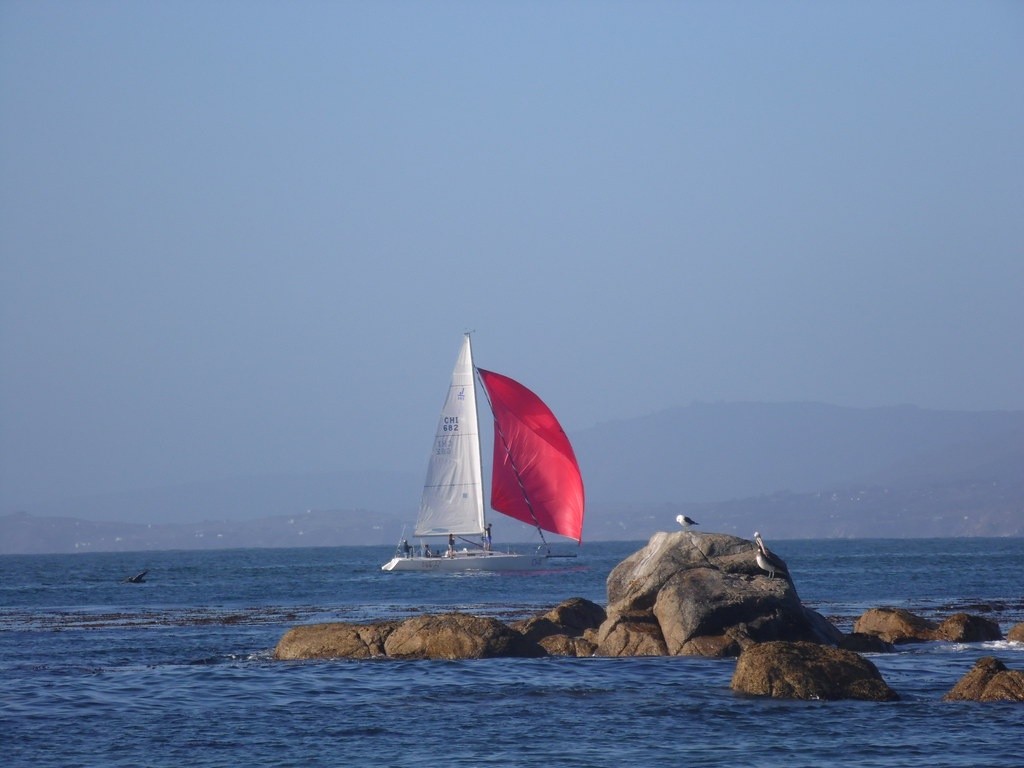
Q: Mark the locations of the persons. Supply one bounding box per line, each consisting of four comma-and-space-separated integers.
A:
403, 539, 412, 559
424, 545, 432, 558
448, 534, 455, 559
485, 523, 492, 551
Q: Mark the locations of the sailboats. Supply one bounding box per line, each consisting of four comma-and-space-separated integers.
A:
379, 327, 586, 574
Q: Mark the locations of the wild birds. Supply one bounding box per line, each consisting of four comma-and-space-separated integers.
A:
754, 532, 768, 558
756, 549, 785, 581
676, 515, 699, 531
129, 571, 147, 582
